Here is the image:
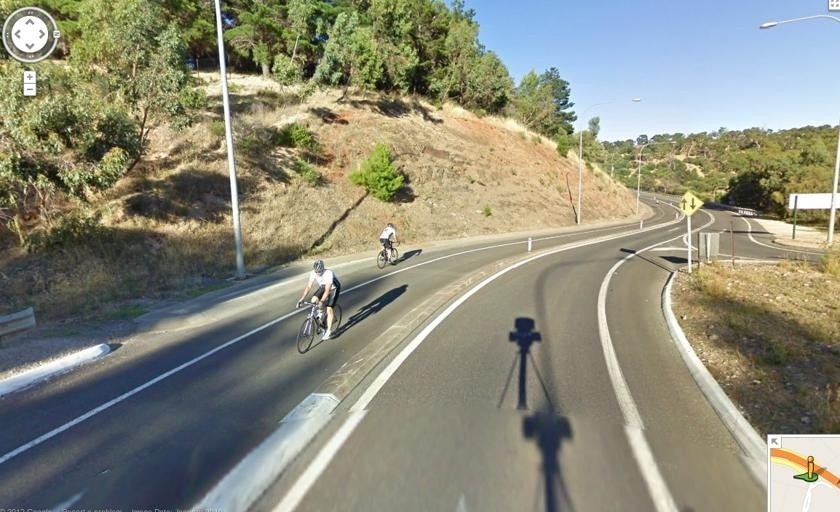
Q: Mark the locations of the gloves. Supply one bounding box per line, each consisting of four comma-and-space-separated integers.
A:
318, 301, 325, 308
296, 299, 304, 308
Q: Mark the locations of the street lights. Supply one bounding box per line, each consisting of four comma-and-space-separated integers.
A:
635, 141, 678, 215
757, 13, 840, 30
576, 98, 641, 223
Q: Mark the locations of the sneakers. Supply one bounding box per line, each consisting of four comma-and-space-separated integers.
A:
316, 313, 322, 320
321, 330, 332, 340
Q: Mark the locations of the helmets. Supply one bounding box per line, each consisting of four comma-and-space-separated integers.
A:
388, 223, 393, 228
314, 261, 324, 272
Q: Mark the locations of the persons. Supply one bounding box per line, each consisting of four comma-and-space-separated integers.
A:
296, 259, 341, 341
379, 222, 397, 263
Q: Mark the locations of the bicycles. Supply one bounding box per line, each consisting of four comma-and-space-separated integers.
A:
376, 241, 402, 270
295, 299, 344, 355
